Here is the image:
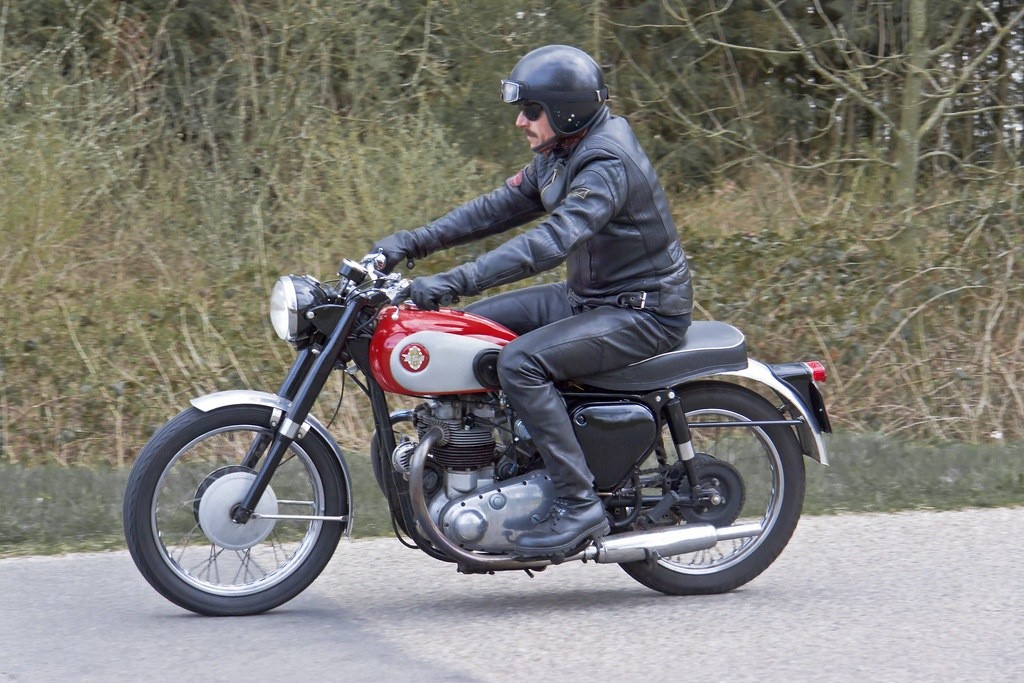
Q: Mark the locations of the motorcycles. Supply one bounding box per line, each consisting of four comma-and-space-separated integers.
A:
122, 254, 830, 615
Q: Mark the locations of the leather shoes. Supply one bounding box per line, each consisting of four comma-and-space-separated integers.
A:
514, 488, 610, 556
503, 499, 555, 531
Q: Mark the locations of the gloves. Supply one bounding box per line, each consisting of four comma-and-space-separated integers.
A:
367, 228, 428, 277
390, 262, 482, 312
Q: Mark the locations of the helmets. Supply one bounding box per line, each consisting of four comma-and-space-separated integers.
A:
509, 44, 610, 136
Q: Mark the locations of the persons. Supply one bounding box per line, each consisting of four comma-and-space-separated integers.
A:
369, 45, 694, 556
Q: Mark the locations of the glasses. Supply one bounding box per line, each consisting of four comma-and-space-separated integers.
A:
499, 79, 525, 104
519, 103, 542, 121
